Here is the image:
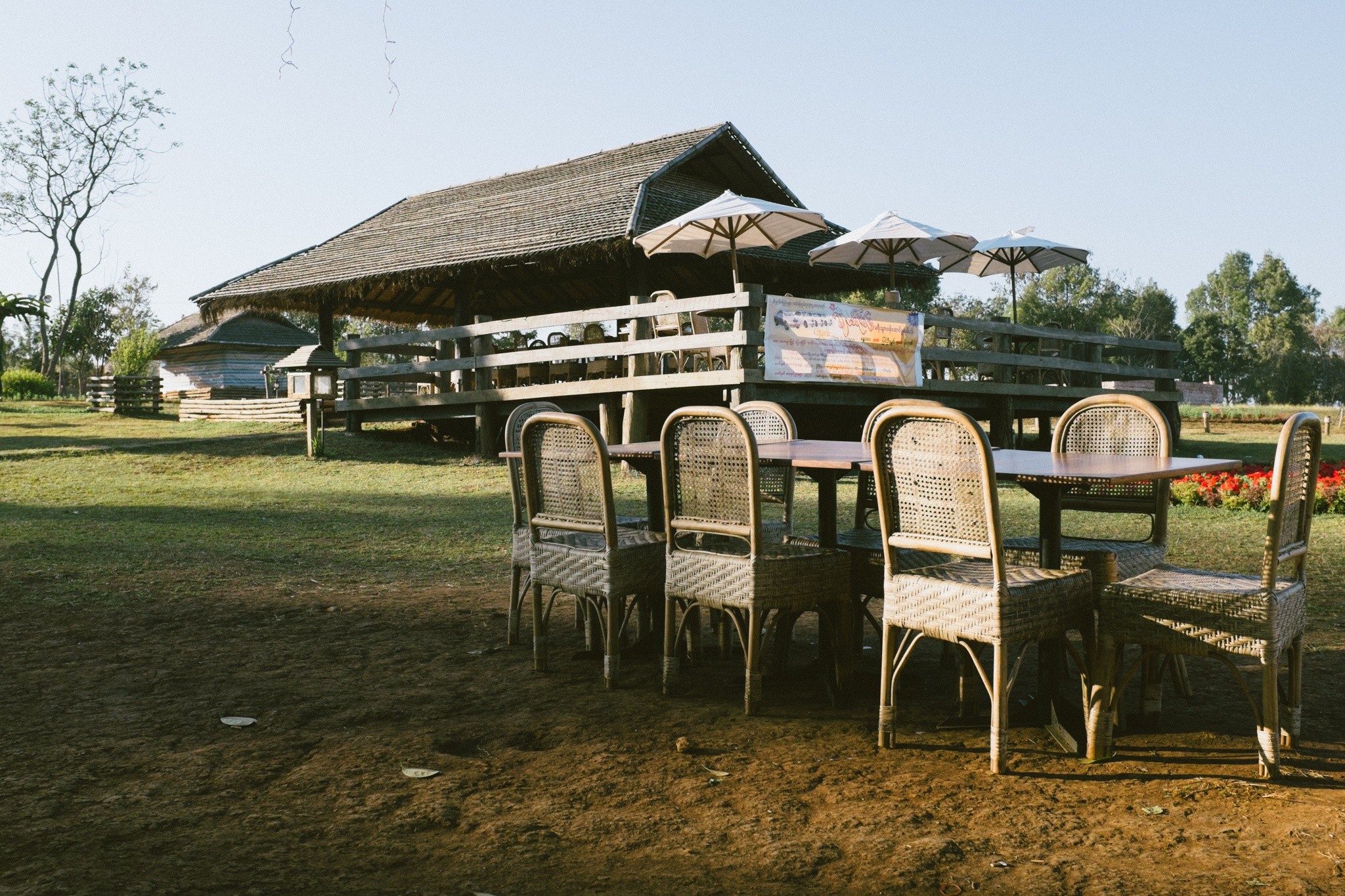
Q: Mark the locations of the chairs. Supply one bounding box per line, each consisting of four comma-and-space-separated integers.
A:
505, 402, 699, 683
660, 401, 854, 718
784, 398, 956, 662
1000, 394, 1192, 696
491, 289, 794, 388
1087, 413, 1322, 779
922, 307, 1071, 386
871, 404, 1091, 771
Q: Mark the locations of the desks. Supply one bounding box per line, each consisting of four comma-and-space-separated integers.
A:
696, 308, 766, 318
500, 440, 1244, 713
983, 334, 1039, 353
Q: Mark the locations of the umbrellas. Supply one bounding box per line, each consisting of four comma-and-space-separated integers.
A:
632, 190, 827, 287
938, 230, 1094, 382
807, 209, 979, 291
